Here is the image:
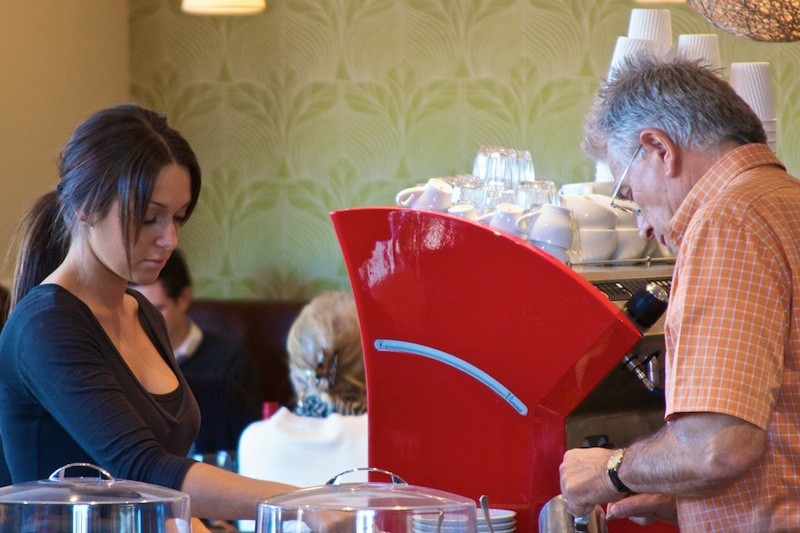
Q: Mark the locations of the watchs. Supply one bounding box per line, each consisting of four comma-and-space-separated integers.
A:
607, 448, 634, 493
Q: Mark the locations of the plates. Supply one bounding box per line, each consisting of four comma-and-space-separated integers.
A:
411, 507, 519, 533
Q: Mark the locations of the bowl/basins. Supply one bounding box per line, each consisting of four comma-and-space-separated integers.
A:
555, 181, 675, 269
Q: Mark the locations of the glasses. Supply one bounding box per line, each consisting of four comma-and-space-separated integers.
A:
610, 144, 643, 221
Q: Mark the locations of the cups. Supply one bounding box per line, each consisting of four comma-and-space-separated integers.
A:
537, 492, 608, 533
395, 146, 582, 267
608, 8, 781, 159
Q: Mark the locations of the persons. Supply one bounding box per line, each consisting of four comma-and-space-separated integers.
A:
558, 49, 800, 533
0, 102, 356, 533
237, 292, 375, 533
125, 247, 263, 456
